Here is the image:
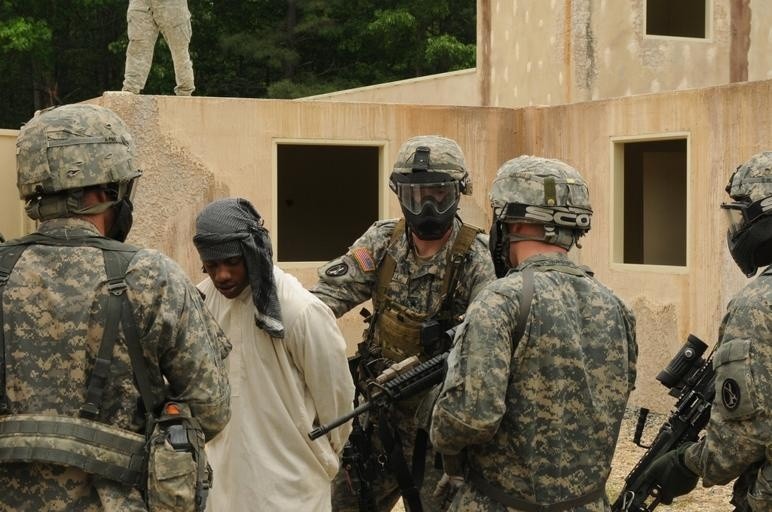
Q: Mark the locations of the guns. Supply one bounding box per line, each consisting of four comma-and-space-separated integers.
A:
341, 424, 409, 512
307, 306, 465, 441
605, 333, 717, 512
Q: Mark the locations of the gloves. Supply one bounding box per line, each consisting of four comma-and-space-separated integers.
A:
642, 440, 699, 506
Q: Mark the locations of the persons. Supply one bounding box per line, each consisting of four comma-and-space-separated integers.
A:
121, 0, 197, 96
192, 196, 356, 512
310, 133, 497, 512
644, 148, 770, 511
0, 102, 233, 512
427, 152, 640, 512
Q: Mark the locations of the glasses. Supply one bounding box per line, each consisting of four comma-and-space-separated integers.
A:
393, 182, 460, 215
722, 200, 768, 241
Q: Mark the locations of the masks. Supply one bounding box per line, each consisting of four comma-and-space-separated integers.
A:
400, 204, 459, 240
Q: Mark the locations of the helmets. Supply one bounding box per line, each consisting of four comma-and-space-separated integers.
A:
389, 136, 473, 214
721, 153, 768, 277
15, 104, 144, 198
490, 155, 594, 234
